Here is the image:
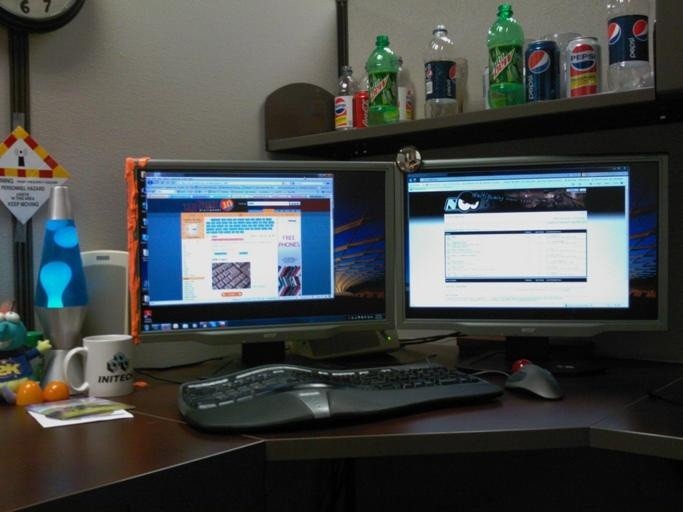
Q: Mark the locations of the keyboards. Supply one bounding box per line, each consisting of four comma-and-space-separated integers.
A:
175, 360, 503, 432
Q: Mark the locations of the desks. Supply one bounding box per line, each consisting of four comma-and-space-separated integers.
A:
0, 344, 683, 512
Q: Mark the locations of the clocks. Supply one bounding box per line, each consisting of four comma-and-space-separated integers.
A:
0, 0, 85, 33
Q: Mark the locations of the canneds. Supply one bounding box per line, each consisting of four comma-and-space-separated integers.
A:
525, 40, 560, 102
566, 36, 601, 99
352, 90, 368, 128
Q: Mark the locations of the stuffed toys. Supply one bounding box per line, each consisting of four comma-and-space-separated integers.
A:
0, 311, 52, 406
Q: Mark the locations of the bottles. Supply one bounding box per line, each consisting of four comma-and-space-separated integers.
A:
333, 33, 415, 131
485, 1, 525, 110
422, 23, 458, 119
23, 331, 44, 385
605, 0, 654, 94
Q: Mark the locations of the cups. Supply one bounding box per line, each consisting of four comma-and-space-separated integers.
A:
62, 334, 134, 398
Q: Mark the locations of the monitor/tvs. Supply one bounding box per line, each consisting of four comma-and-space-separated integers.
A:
125, 157, 391, 379
392, 153, 668, 370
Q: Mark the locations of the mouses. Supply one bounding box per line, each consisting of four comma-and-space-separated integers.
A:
505, 357, 565, 400
